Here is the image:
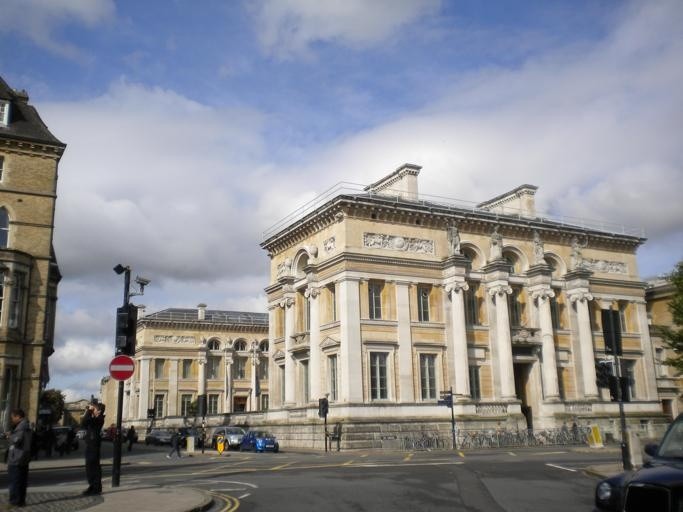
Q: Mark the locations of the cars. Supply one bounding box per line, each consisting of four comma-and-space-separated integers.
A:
74, 426, 138, 444
593, 410, 683, 511
144, 431, 172, 447
49, 426, 80, 451
239, 430, 279, 454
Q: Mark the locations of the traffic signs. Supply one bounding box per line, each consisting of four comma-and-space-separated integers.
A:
436, 389, 452, 407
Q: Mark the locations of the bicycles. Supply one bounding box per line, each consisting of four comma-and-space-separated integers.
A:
404, 423, 588, 450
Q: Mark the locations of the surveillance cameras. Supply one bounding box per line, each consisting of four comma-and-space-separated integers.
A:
135, 276, 152, 286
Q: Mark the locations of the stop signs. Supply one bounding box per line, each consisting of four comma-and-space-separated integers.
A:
109, 355, 135, 381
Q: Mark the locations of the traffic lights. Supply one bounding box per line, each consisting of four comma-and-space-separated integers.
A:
112, 308, 133, 350
595, 361, 606, 385
320, 399, 328, 413
607, 374, 619, 403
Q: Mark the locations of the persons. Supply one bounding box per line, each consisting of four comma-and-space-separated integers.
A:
24, 419, 80, 460
167, 431, 182, 459
110, 422, 116, 440
80, 401, 106, 495
5, 408, 34, 508
561, 421, 569, 439
126, 425, 135, 452
571, 422, 578, 436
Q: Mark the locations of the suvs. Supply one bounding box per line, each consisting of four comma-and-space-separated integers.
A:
210, 427, 247, 451
170, 428, 204, 447
